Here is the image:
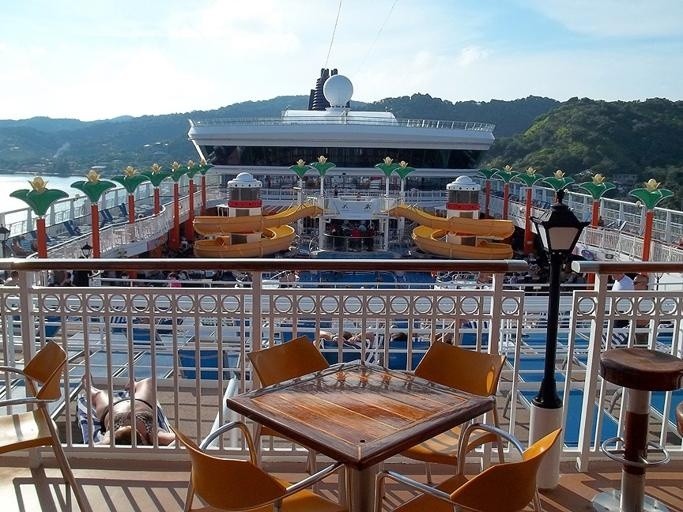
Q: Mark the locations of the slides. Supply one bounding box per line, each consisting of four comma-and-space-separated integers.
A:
380, 203, 515, 259
191, 202, 326, 259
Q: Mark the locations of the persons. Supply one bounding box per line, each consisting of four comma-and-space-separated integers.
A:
509, 258, 594, 293
608, 273, 633, 328
631, 272, 651, 328
318, 328, 453, 349
277, 270, 296, 289
81, 373, 176, 447
0, 235, 219, 288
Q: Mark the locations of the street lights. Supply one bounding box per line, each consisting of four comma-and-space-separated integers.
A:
395, 159, 414, 245
9, 158, 218, 288
524, 188, 589, 491
311, 154, 336, 251
477, 162, 617, 289
289, 155, 311, 245
376, 155, 399, 251
636, 178, 675, 274
